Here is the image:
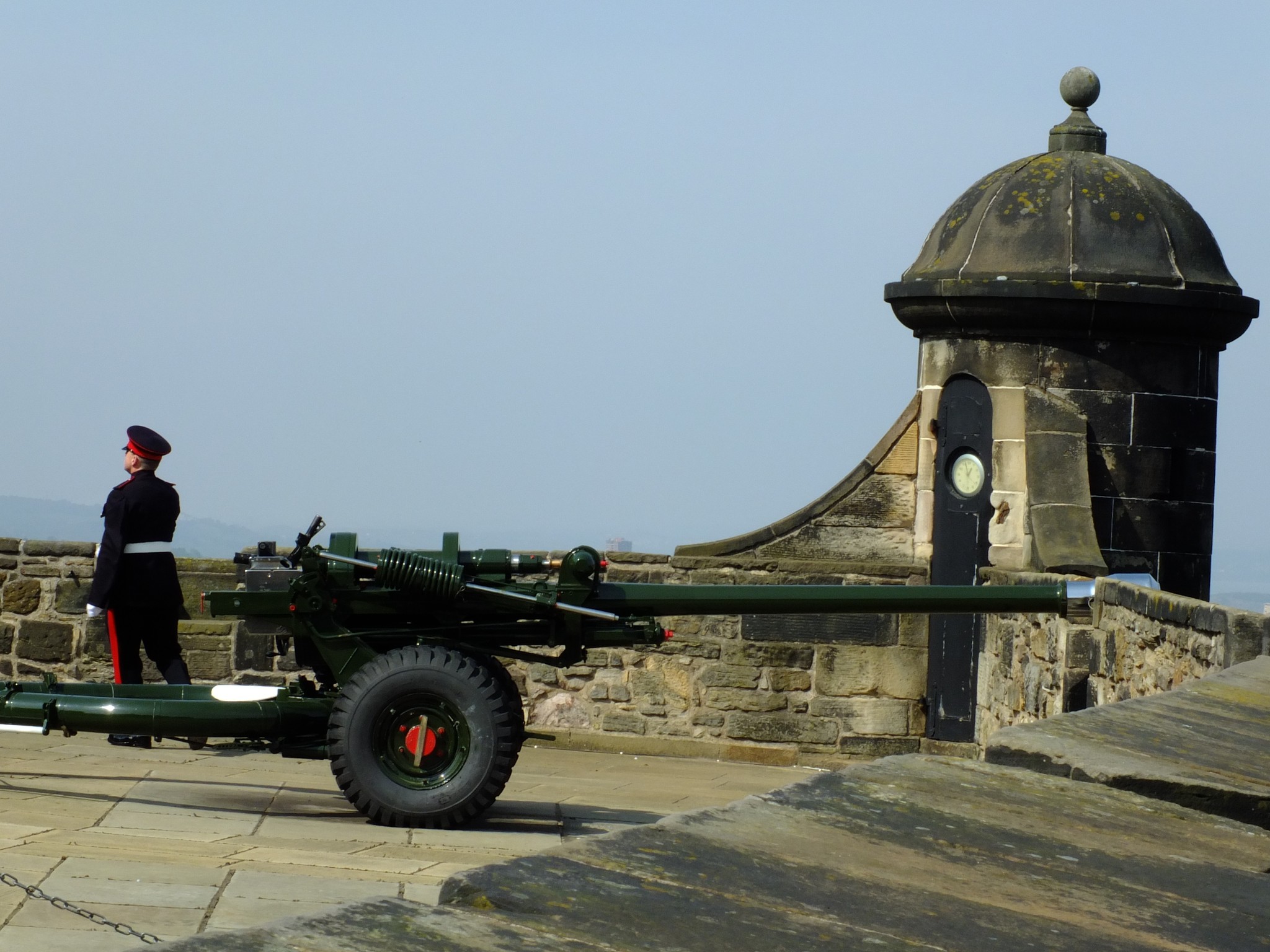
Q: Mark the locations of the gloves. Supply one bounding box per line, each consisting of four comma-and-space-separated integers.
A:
86, 603, 103, 618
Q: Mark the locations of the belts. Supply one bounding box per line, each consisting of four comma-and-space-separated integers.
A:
95, 541, 172, 557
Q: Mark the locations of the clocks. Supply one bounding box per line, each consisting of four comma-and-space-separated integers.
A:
953, 452, 988, 499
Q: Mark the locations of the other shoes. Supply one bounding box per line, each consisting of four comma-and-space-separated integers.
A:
107, 734, 151, 749
187, 736, 208, 750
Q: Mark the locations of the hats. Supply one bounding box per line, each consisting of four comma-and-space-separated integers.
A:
121, 425, 172, 460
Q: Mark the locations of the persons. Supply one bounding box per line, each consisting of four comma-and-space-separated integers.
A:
85, 425, 209, 751
